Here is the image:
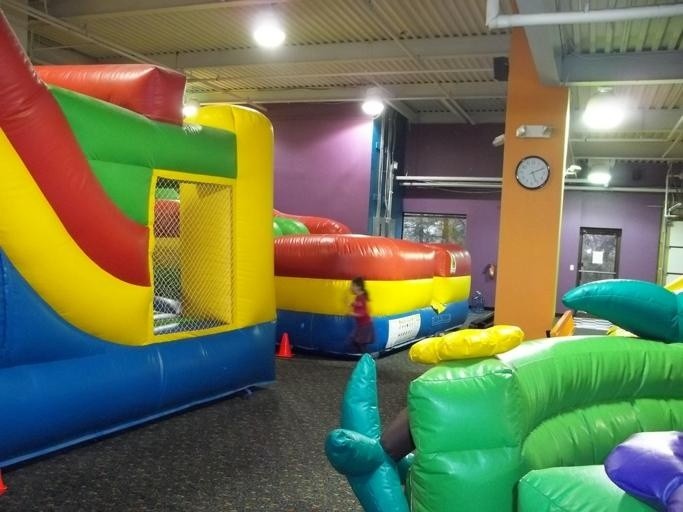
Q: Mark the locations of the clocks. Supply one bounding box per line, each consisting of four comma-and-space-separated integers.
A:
515, 155, 551, 190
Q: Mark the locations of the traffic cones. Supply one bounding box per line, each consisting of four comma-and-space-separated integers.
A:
276, 332, 294, 358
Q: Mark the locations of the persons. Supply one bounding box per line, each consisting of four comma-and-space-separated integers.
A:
344, 276, 375, 356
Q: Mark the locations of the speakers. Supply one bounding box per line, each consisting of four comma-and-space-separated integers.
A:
493, 56, 508, 82
